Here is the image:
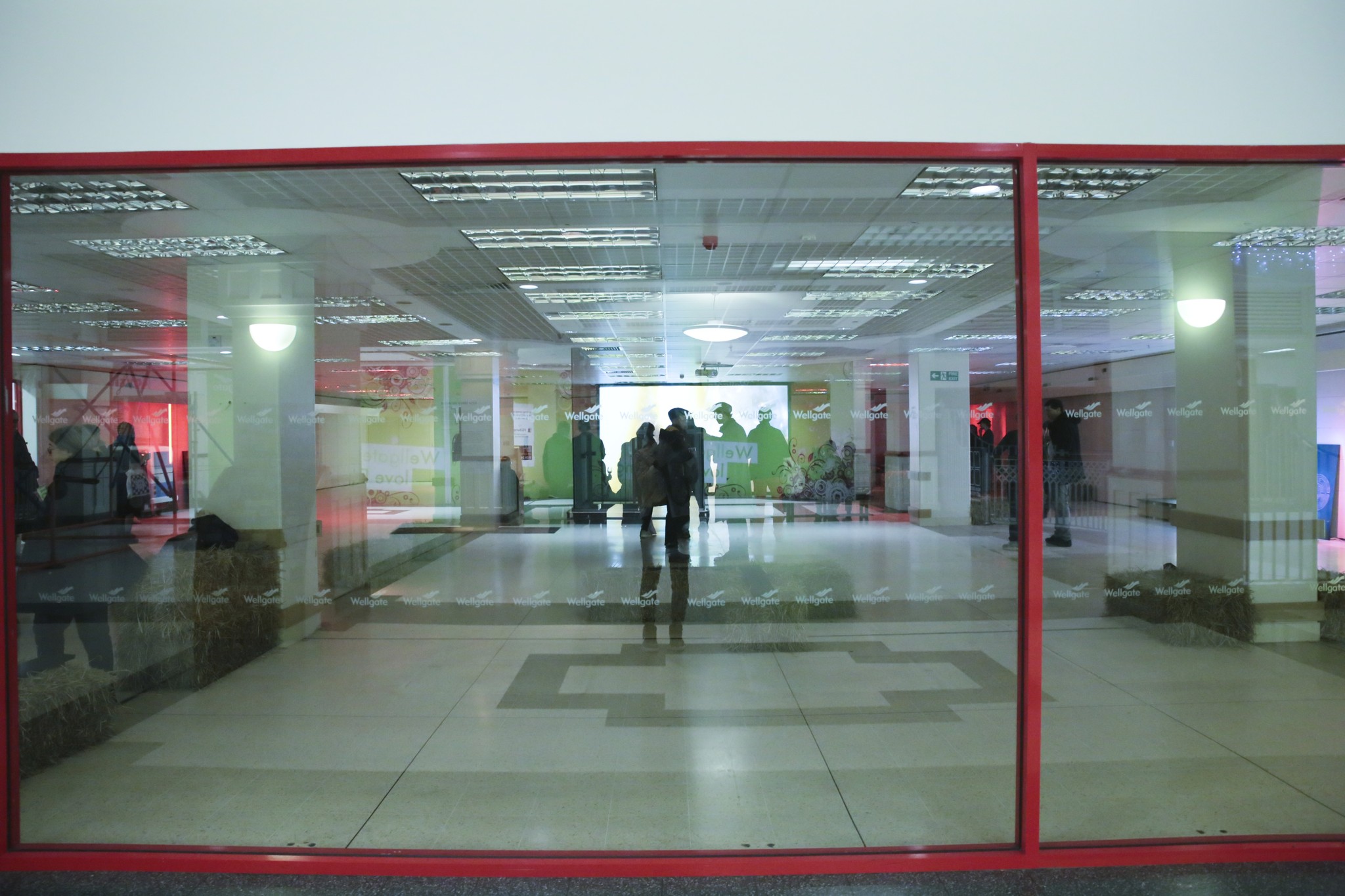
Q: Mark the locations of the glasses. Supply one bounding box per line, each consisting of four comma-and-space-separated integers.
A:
48, 445, 59, 454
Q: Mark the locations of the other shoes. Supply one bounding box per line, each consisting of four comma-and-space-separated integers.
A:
676, 530, 690, 538
669, 552, 690, 562
122, 534, 140, 543
699, 508, 709, 518
1002, 540, 1018, 550
638, 529, 652, 536
1045, 536, 1072, 547
664, 547, 678, 553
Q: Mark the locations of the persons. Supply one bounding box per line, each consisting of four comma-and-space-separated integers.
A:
977, 418, 994, 495
813, 440, 867, 523
632, 424, 699, 653
543, 403, 794, 572
14, 410, 149, 675
1043, 397, 1085, 547
991, 413, 1020, 551
970, 424, 983, 496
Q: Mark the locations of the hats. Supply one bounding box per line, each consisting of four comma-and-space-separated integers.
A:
49, 425, 100, 454
710, 402, 732, 413
977, 418, 990, 424
